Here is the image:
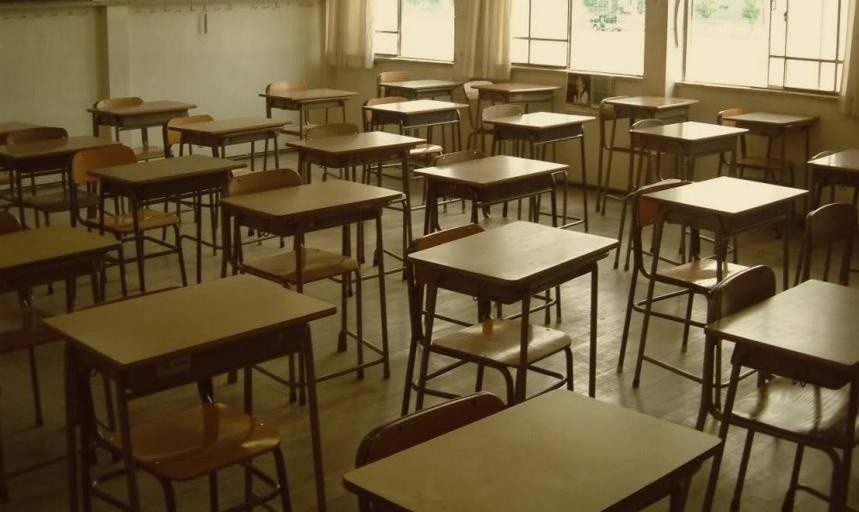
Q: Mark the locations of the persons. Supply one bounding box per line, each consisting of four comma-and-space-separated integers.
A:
572, 77, 590, 106
